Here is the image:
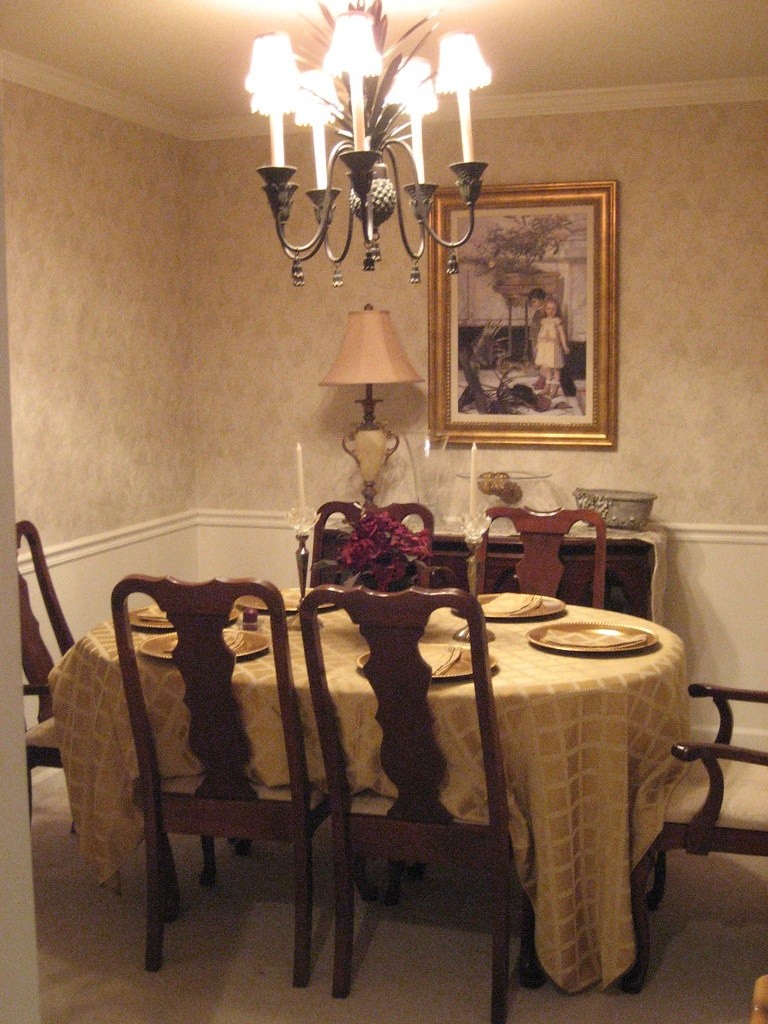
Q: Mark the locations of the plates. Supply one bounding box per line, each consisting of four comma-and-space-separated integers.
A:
451, 593, 566, 618
357, 643, 497, 678
140, 629, 270, 659
128, 607, 240, 628
238, 588, 335, 611
526, 622, 658, 652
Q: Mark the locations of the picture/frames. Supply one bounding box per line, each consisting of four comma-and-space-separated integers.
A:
428, 180, 618, 446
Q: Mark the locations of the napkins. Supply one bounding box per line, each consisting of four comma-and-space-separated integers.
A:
281, 589, 313, 608
417, 642, 462, 676
164, 632, 245, 653
136, 603, 169, 622
539, 629, 648, 649
481, 593, 542, 616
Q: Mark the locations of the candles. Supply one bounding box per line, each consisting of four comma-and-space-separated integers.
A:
470, 442, 478, 518
296, 443, 306, 519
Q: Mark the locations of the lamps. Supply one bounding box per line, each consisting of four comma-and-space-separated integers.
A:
244, 0, 492, 287
318, 302, 426, 510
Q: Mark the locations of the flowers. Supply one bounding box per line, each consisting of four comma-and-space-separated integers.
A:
337, 511, 433, 593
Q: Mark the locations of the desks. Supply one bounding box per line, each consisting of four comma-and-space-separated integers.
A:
47, 587, 694, 994
309, 527, 669, 627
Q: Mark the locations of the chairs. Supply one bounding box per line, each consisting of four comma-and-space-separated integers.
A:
15, 521, 78, 835
300, 586, 548, 1024
310, 503, 434, 588
617, 682, 768, 993
111, 573, 330, 989
477, 507, 607, 611
